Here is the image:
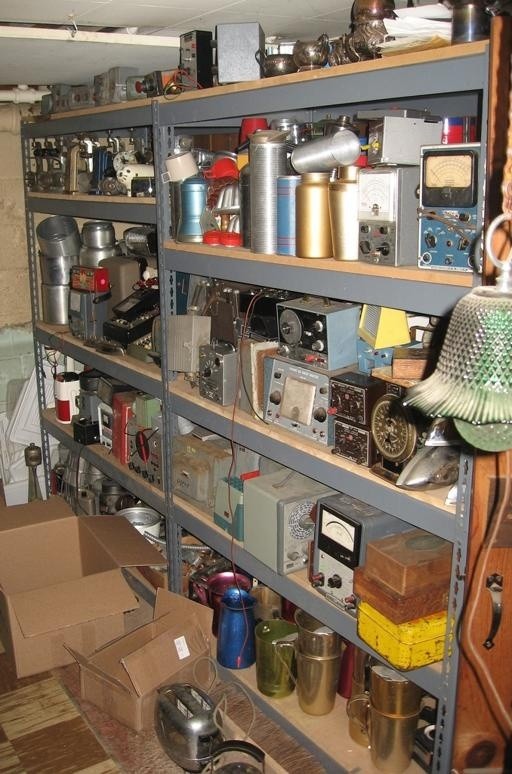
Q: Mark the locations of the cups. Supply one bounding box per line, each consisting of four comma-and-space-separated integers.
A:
346, 691, 420, 772
451, 3, 486, 43
160, 151, 199, 183
254, 619, 298, 699
370, 664, 423, 717
293, 607, 344, 658
273, 640, 343, 716
345, 645, 372, 749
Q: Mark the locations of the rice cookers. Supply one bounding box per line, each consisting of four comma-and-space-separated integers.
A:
115, 506, 165, 544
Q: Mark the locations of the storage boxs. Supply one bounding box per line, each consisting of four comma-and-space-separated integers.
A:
1, 485, 172, 683
50, 582, 227, 737
349, 564, 451, 627
363, 527, 454, 598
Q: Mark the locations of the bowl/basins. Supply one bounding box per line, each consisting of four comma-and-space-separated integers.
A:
34, 213, 122, 285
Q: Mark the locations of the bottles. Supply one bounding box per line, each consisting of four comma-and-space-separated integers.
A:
238, 113, 359, 263
28, 465, 43, 503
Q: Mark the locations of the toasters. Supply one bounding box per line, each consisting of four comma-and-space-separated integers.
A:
155, 682, 225, 774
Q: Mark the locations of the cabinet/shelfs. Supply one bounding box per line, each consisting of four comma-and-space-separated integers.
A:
15, 8, 509, 774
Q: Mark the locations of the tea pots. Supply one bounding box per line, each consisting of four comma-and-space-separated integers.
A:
192, 571, 251, 659
217, 587, 257, 670
